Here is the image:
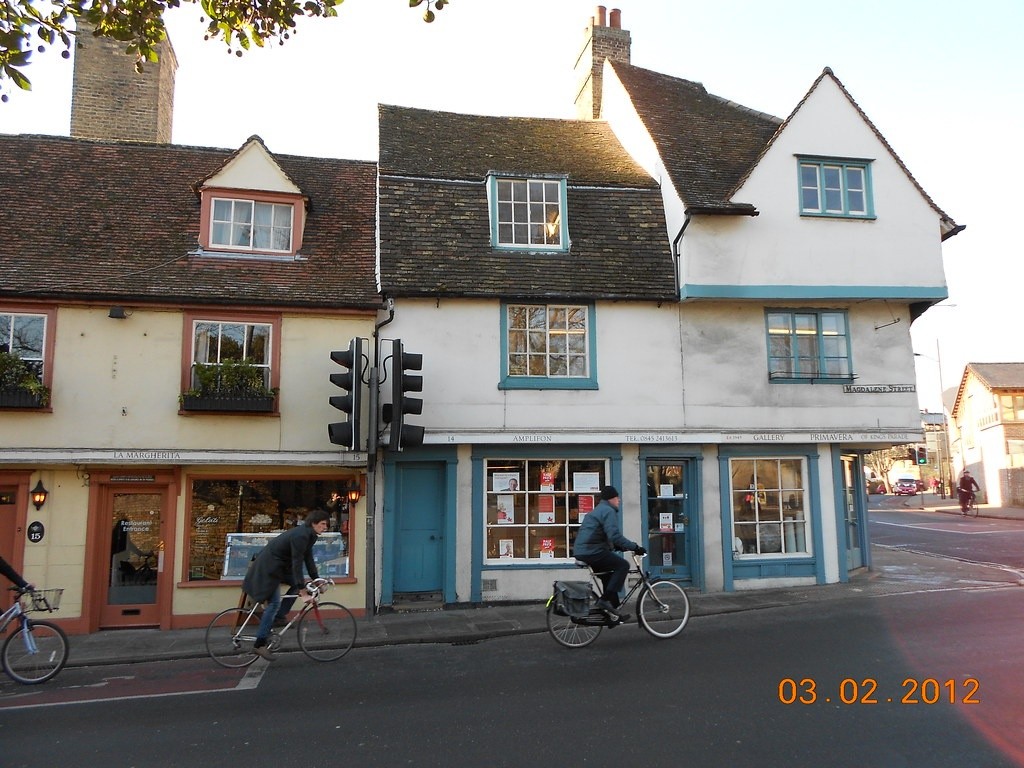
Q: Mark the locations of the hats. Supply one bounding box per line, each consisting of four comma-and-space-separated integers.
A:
963, 470, 969, 473
600, 485, 618, 499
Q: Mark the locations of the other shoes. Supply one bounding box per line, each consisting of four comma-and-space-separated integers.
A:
594, 596, 617, 613
608, 615, 630, 629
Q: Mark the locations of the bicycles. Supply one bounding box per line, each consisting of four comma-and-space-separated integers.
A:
0, 585, 68, 685
133, 552, 158, 586
545, 552, 691, 649
960, 490, 979, 518
204, 578, 357, 669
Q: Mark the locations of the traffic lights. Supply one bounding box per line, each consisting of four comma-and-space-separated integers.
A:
326, 336, 362, 453
388, 337, 426, 454
908, 448, 917, 465
916, 444, 928, 466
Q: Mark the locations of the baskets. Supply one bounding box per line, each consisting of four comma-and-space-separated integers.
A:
29, 588, 64, 610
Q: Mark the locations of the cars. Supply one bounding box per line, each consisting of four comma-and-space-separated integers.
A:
894, 479, 917, 496
876, 484, 894, 495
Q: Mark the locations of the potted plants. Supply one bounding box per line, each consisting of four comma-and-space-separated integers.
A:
0, 349, 50, 408
181, 356, 279, 412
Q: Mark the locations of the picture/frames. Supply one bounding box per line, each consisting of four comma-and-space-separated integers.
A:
192, 566, 205, 577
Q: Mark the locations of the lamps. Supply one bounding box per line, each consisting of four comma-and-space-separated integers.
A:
30, 481, 48, 510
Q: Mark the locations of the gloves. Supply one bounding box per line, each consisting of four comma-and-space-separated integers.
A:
632, 542, 647, 557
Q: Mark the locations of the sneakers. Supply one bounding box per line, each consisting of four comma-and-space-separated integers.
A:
271, 616, 294, 629
251, 644, 273, 662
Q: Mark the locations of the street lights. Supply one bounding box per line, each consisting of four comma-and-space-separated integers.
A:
931, 302, 958, 499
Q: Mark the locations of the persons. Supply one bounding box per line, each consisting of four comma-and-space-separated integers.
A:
574, 486, 646, 630
961, 470, 980, 516
0, 556, 34, 590
243, 511, 330, 661
501, 479, 519, 491
931, 476, 937, 495
